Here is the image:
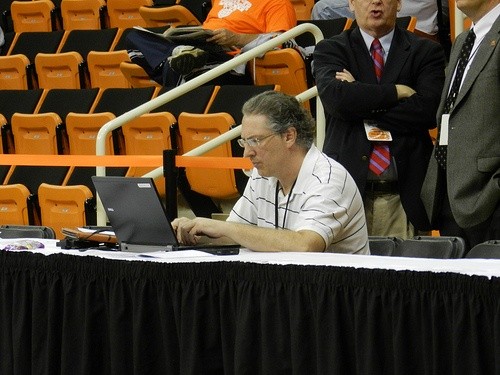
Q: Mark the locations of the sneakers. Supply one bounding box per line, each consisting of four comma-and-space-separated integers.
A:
168, 45, 209, 75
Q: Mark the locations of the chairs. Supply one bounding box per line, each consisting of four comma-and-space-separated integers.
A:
0, 0, 500, 258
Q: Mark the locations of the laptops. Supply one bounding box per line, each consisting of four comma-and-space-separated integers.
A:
91, 176, 241, 254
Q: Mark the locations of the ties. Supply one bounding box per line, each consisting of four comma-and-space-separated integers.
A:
369, 38, 390, 177
435, 28, 476, 171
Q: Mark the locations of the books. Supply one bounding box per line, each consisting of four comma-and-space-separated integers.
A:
61, 230, 118, 242
132, 25, 237, 52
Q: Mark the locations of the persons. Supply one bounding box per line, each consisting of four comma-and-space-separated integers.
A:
310, 0, 356, 22
314, 0, 445, 239
152, 0, 212, 25
172, 90, 371, 256
395, 0, 438, 40
121, 0, 298, 152
419, 0, 500, 257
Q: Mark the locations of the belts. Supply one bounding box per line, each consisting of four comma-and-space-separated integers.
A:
375, 179, 399, 193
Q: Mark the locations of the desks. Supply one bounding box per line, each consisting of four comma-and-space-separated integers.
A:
0, 238, 500, 375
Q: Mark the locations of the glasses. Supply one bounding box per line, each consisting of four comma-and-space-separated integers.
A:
237, 131, 280, 147
